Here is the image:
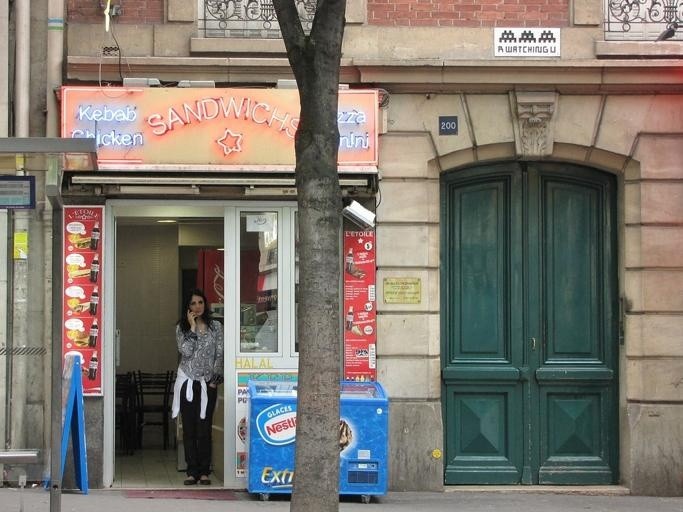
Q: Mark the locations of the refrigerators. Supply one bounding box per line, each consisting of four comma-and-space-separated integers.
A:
247, 380, 390, 504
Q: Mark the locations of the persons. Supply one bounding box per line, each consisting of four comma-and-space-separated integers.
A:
176, 290, 224, 485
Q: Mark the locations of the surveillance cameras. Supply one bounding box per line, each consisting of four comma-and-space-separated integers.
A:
342, 200, 376, 231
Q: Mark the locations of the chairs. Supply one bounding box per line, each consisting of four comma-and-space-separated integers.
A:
115, 370, 177, 449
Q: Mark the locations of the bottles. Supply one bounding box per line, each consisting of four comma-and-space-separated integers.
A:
89, 318, 98, 347
90, 221, 100, 250
90, 253, 99, 282
346, 306, 353, 330
89, 286, 99, 316
346, 247, 353, 274
88, 351, 98, 381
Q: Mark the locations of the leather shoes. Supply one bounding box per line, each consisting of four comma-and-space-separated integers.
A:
184, 479, 210, 485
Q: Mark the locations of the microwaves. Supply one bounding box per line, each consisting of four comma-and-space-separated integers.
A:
211, 303, 257, 326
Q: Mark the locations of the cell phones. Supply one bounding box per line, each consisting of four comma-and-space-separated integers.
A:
188, 307, 197, 321
